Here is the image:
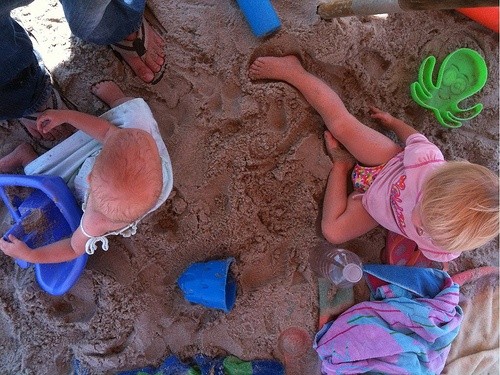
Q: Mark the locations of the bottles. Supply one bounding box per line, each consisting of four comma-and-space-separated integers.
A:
308, 243, 363, 290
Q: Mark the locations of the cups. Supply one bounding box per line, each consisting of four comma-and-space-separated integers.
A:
177, 256, 238, 313
387, 228, 431, 267
236, 0, 282, 38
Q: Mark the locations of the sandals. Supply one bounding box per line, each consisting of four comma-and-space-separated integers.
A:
15, 91, 76, 151
109, 15, 167, 84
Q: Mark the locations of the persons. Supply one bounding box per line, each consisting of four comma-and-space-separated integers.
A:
249, 55, 500, 262
0, 0, 168, 151
0, 81, 174, 264
